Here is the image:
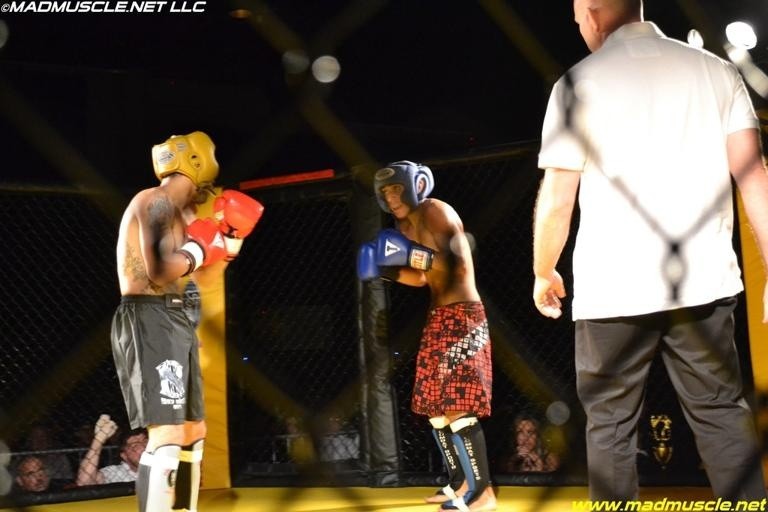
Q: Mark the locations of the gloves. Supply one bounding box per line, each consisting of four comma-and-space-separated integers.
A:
210, 189, 265, 262
375, 228, 434, 274
358, 241, 400, 287
175, 215, 229, 278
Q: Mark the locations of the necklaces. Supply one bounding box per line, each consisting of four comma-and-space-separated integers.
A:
3, 387, 703, 487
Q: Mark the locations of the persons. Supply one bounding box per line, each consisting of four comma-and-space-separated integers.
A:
532, 1, 767, 512
111, 126, 265, 512
355, 155, 504, 512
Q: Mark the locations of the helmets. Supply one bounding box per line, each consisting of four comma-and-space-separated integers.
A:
152, 131, 219, 204
373, 161, 436, 215
